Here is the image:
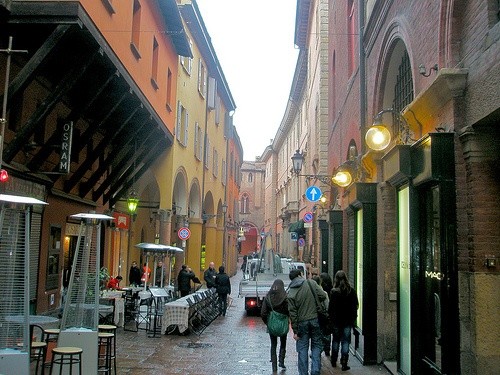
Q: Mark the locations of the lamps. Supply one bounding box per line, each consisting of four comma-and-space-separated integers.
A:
364, 109, 409, 151
202, 201, 229, 221
332, 160, 359, 187
291, 149, 331, 186
127, 138, 141, 218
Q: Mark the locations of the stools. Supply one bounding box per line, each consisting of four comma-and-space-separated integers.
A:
30, 324, 117, 375
124, 289, 153, 333
186, 287, 221, 336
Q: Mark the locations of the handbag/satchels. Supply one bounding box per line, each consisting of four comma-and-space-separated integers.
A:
268, 311, 289, 336
318, 313, 331, 336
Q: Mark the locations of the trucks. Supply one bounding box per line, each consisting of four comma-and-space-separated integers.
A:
237, 256, 307, 317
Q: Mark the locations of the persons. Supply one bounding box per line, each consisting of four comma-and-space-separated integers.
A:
105, 252, 256, 317
261, 268, 360, 375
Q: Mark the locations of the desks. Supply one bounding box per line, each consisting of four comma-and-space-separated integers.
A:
100, 296, 125, 328
6, 315, 59, 342
70, 304, 114, 320
108, 291, 127, 298
122, 287, 144, 295
161, 288, 212, 335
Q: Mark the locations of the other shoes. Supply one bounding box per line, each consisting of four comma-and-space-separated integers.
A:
331, 361, 336, 367
272, 366, 277, 371
219, 312, 222, 316
279, 363, 285, 369
223, 312, 225, 317
342, 365, 350, 370
325, 350, 330, 357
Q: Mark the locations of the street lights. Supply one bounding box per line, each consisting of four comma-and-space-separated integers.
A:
125, 189, 140, 288
